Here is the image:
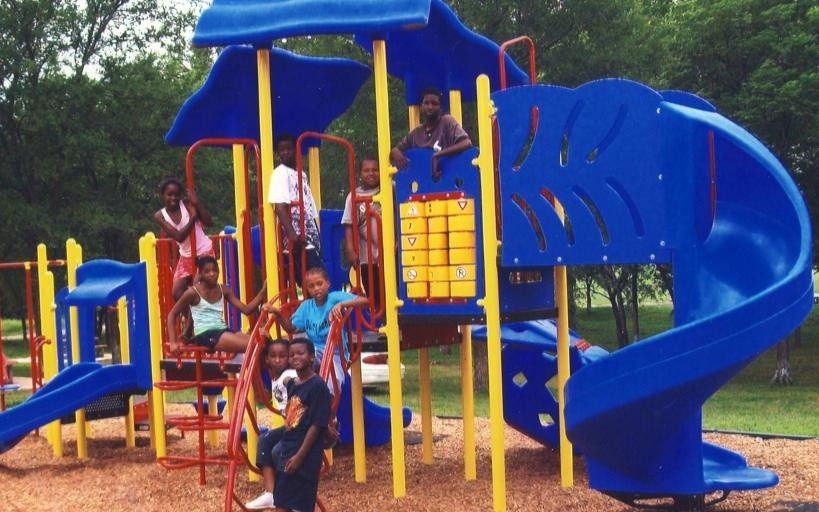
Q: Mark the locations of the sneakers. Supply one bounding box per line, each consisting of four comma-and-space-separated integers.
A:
245, 492, 276, 509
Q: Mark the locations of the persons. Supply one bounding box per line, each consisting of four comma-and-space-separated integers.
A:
273, 336, 329, 512
340, 158, 382, 313
154, 177, 213, 345
260, 267, 369, 404
244, 338, 298, 511
267, 135, 324, 288
167, 256, 267, 357
390, 86, 473, 183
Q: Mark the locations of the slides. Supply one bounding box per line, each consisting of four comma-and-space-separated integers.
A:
260, 367, 412, 444
564, 102, 815, 512
0, 362, 149, 451
